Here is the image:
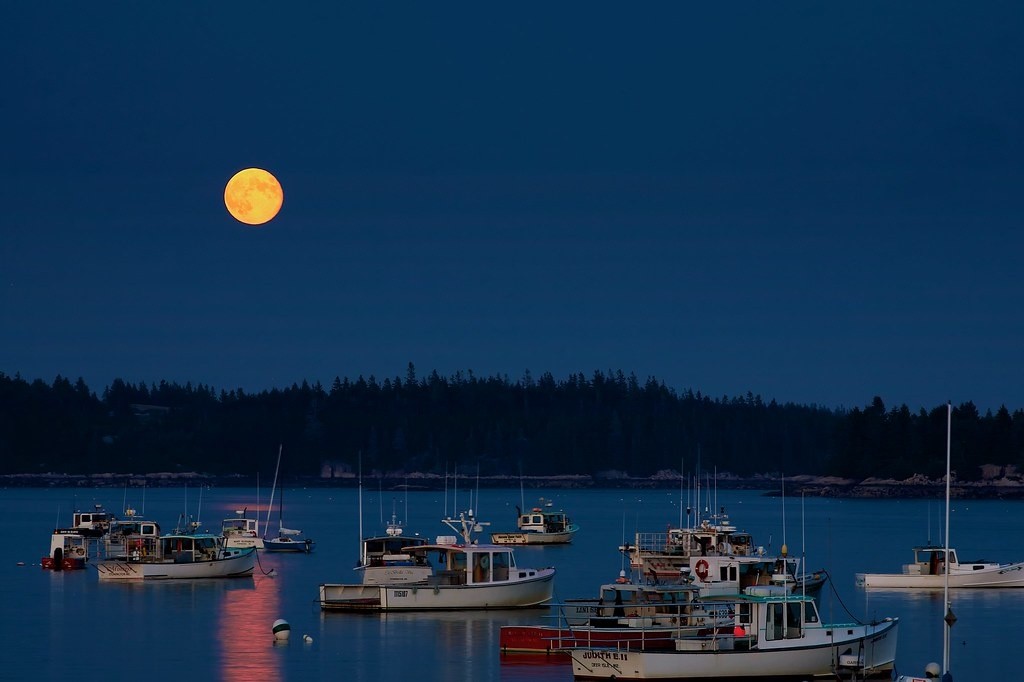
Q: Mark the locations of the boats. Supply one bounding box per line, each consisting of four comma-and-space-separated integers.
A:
358, 461, 435, 587
312, 460, 557, 614
620, 451, 768, 587
854, 498, 1024, 588
563, 463, 828, 629
488, 495, 580, 547
497, 470, 899, 682
36, 477, 315, 585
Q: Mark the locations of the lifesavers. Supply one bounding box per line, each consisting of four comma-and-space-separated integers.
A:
695, 560, 709, 579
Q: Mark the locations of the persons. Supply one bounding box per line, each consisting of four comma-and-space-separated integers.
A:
700, 540, 707, 555
132, 547, 140, 562
626, 611, 640, 617
648, 567, 660, 586
547, 519, 553, 531
124, 507, 130, 517
237, 521, 244, 529
555, 520, 562, 532
201, 544, 207, 554
624, 540, 629, 549
129, 507, 135, 515
